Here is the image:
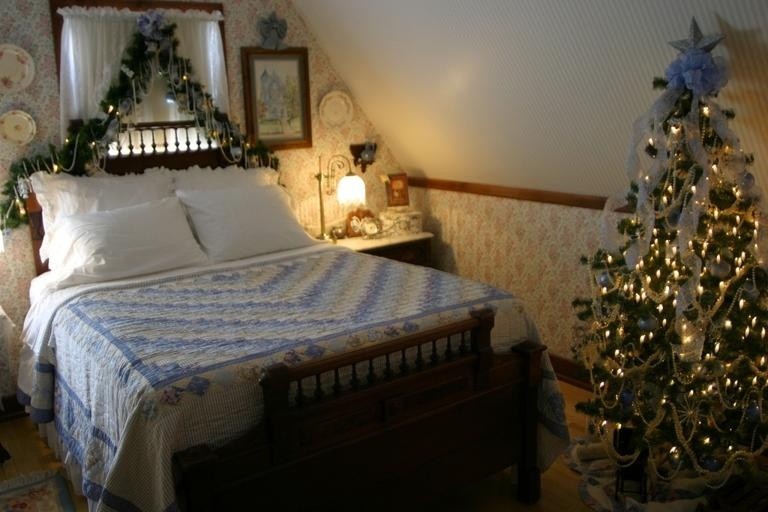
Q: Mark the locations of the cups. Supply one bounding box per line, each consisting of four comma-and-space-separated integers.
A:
331, 225, 346, 239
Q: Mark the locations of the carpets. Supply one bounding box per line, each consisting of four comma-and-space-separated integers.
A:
3, 466, 76, 512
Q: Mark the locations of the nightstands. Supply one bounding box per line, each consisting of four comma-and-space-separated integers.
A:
323, 230, 434, 270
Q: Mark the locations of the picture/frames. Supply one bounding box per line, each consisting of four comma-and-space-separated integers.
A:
240, 44, 313, 150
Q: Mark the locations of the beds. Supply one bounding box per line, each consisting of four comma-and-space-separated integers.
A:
22, 148, 549, 512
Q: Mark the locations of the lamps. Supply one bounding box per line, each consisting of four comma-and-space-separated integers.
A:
310, 155, 366, 208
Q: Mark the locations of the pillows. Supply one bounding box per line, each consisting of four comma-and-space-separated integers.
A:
26, 161, 318, 294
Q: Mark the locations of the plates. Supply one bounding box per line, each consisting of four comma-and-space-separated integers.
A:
0, 110, 36, 147
318, 91, 353, 130
0, 42, 35, 93
